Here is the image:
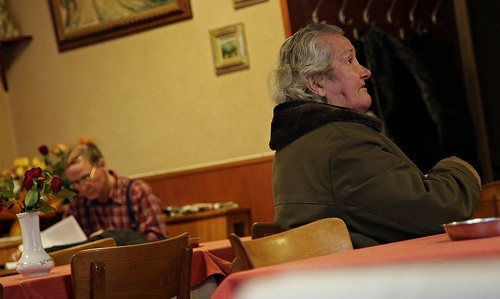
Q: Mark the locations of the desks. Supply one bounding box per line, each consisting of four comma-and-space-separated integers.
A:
211, 231, 500, 299
0, 236, 252, 299
164, 207, 251, 243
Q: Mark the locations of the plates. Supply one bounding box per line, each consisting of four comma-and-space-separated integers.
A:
442, 218, 500, 241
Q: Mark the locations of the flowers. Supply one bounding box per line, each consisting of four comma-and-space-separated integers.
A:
0, 142, 79, 215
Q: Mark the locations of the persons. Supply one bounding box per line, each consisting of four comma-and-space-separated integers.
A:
8, 143, 168, 261
268, 23, 482, 249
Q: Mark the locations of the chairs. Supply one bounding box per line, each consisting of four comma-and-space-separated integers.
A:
0, 218, 352, 299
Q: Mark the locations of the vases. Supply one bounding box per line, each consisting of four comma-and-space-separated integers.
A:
16, 212, 55, 279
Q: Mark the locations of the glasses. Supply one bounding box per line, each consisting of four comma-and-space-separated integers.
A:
69, 165, 97, 190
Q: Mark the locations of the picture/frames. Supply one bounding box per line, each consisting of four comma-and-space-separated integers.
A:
208, 22, 250, 78
47, 1, 194, 53
